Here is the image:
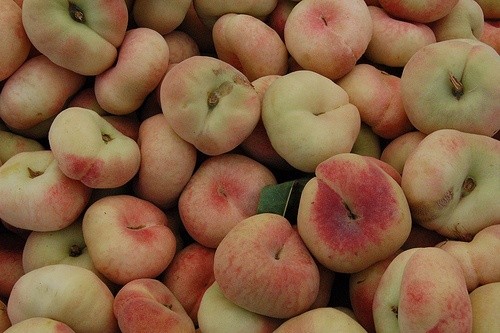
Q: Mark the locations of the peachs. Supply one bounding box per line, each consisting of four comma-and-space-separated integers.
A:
0, 0, 500, 333
